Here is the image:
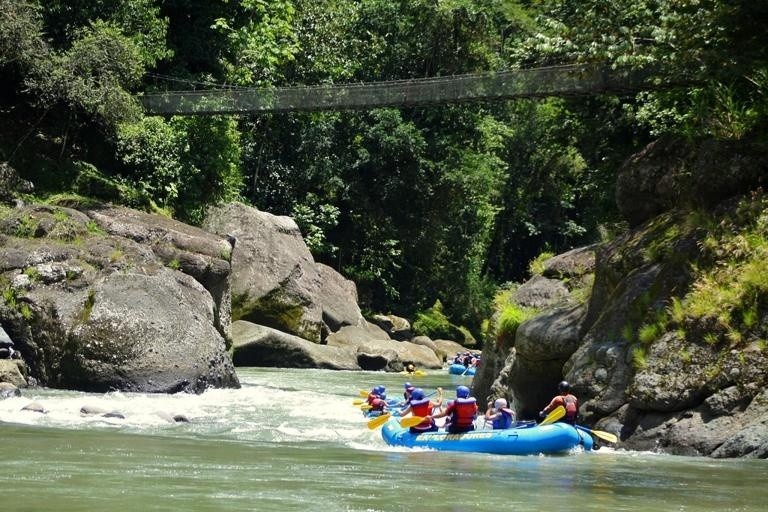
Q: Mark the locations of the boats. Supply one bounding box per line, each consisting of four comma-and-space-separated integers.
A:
381, 411, 594, 456
448, 362, 477, 375
363, 395, 432, 424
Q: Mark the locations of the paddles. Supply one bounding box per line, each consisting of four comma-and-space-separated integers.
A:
539, 410, 618, 443
537, 405, 567, 427
353, 388, 403, 411
367, 390, 439, 430
400, 415, 425, 428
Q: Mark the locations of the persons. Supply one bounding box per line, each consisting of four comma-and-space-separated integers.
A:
367, 351, 578, 433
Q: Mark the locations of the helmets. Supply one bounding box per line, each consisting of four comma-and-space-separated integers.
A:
557, 380, 570, 392
494, 398, 507, 411
404, 381, 425, 399
456, 385, 470, 398
372, 384, 389, 400
